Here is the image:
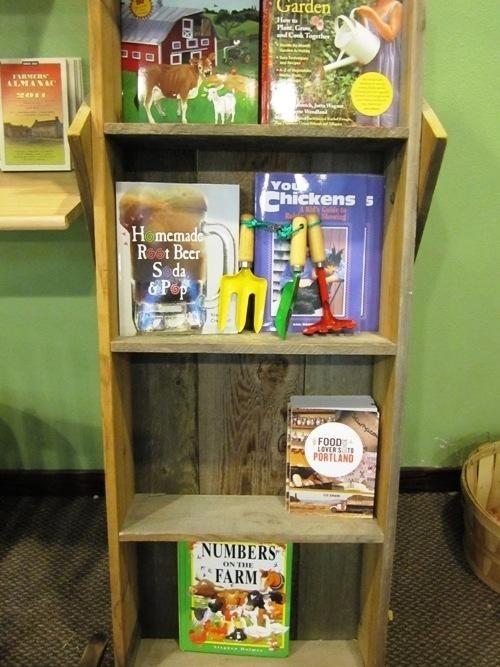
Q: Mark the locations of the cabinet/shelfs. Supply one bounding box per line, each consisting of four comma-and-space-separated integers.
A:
88, 0, 425, 667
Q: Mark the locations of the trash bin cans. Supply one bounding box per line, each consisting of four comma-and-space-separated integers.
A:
459, 440, 500, 593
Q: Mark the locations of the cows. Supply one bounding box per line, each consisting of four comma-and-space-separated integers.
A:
134, 53, 216, 125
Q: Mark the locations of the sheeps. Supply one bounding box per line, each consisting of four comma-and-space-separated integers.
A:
203, 84, 236, 124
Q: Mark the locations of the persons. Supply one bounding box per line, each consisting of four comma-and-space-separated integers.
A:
355, 1, 406, 127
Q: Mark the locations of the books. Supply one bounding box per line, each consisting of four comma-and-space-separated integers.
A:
118, 1, 260, 125
115, 180, 242, 339
260, 1, 404, 129
284, 393, 379, 520
1, 57, 84, 172
252, 169, 387, 333
177, 539, 294, 658
